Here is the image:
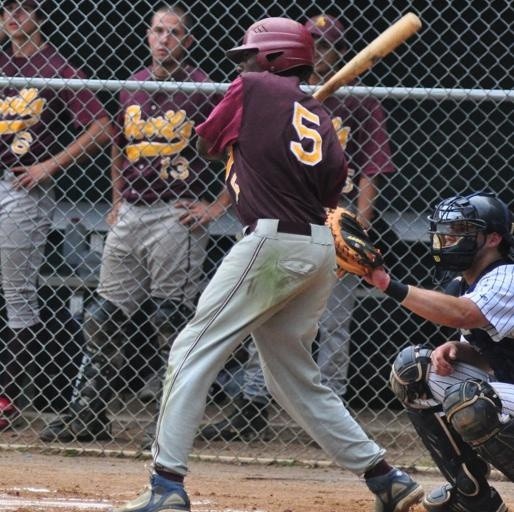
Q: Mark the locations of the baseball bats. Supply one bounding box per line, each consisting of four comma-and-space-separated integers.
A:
313, 13, 422, 104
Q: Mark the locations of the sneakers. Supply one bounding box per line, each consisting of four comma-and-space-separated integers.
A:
0, 395, 21, 432
198, 398, 268, 442
140, 375, 161, 401
142, 409, 157, 449
112, 466, 191, 510
409, 485, 509, 512
358, 468, 423, 512
40, 411, 111, 441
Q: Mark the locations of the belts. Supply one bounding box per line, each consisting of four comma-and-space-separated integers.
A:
247, 218, 313, 237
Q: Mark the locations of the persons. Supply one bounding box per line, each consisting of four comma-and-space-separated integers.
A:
0, 1, 114, 427
322, 190, 514, 512
196, 14, 398, 448
118, 15, 425, 511
35, 4, 229, 445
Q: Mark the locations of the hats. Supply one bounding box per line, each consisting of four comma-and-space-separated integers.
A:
305, 15, 348, 47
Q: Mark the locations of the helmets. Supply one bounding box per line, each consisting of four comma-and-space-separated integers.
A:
224, 17, 315, 74
427, 192, 513, 270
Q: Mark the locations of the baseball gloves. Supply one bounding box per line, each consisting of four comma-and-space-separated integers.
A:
324, 206, 383, 278
